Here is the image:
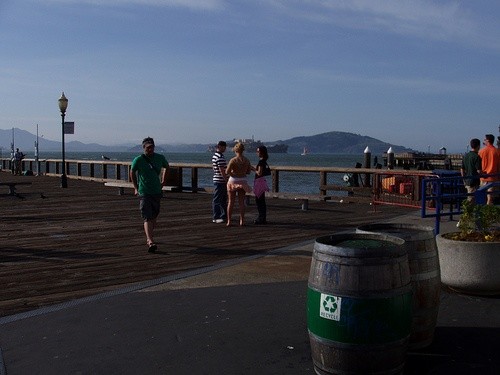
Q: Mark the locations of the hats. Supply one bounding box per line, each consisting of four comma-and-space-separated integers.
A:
143, 138, 154, 149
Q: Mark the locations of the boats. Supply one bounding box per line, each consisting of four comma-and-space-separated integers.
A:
207, 138, 289, 154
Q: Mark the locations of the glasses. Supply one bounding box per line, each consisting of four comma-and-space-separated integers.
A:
484, 138, 488, 141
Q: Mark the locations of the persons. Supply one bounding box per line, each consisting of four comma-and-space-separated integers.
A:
212, 140, 272, 228
14, 148, 22, 176
456, 134, 500, 228
130, 137, 169, 253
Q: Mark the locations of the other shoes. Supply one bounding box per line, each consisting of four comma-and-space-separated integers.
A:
456, 218, 464, 227
253, 219, 266, 224
147, 244, 157, 252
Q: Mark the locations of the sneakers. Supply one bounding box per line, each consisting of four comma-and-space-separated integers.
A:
213, 219, 224, 223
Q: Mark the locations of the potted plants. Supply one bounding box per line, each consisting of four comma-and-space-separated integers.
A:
437, 199, 500, 296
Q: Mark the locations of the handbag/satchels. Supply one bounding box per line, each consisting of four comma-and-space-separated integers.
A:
262, 162, 271, 176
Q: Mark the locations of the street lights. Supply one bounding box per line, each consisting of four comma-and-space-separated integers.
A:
56, 91, 68, 189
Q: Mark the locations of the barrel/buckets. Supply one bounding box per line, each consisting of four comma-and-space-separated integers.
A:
357, 223, 441, 348
306, 233, 409, 375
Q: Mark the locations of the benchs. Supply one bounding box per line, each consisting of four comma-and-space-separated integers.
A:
0, 181, 32, 196
105, 181, 178, 196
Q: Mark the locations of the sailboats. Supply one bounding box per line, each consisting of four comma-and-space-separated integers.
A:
301, 147, 310, 156
